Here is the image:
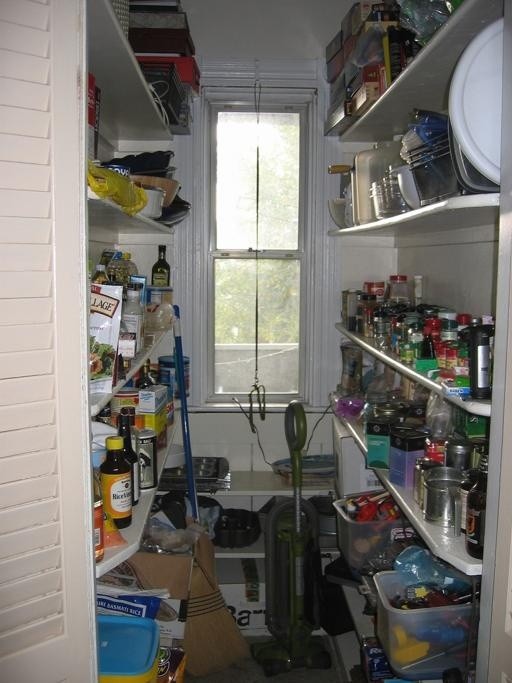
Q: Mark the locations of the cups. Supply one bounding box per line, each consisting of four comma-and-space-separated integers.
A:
422, 467, 466, 538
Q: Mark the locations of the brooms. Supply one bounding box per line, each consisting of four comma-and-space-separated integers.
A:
172, 302, 268, 682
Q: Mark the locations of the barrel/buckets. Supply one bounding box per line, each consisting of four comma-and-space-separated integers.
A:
350, 140, 408, 225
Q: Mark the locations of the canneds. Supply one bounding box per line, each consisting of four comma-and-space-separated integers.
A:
364, 281, 384, 303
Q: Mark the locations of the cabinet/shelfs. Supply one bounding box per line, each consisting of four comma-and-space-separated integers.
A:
87, 1, 293, 683
293, 0, 512, 683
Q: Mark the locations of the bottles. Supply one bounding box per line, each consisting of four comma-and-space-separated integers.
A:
391, 314, 408, 355
466, 452, 489, 559
99, 406, 140, 528
468, 325, 491, 400
91, 252, 139, 284
122, 283, 146, 354
342, 288, 377, 338
412, 275, 423, 312
389, 275, 410, 302
413, 457, 432, 508
136, 362, 153, 389
445, 440, 471, 471
151, 244, 170, 287
421, 327, 435, 359
